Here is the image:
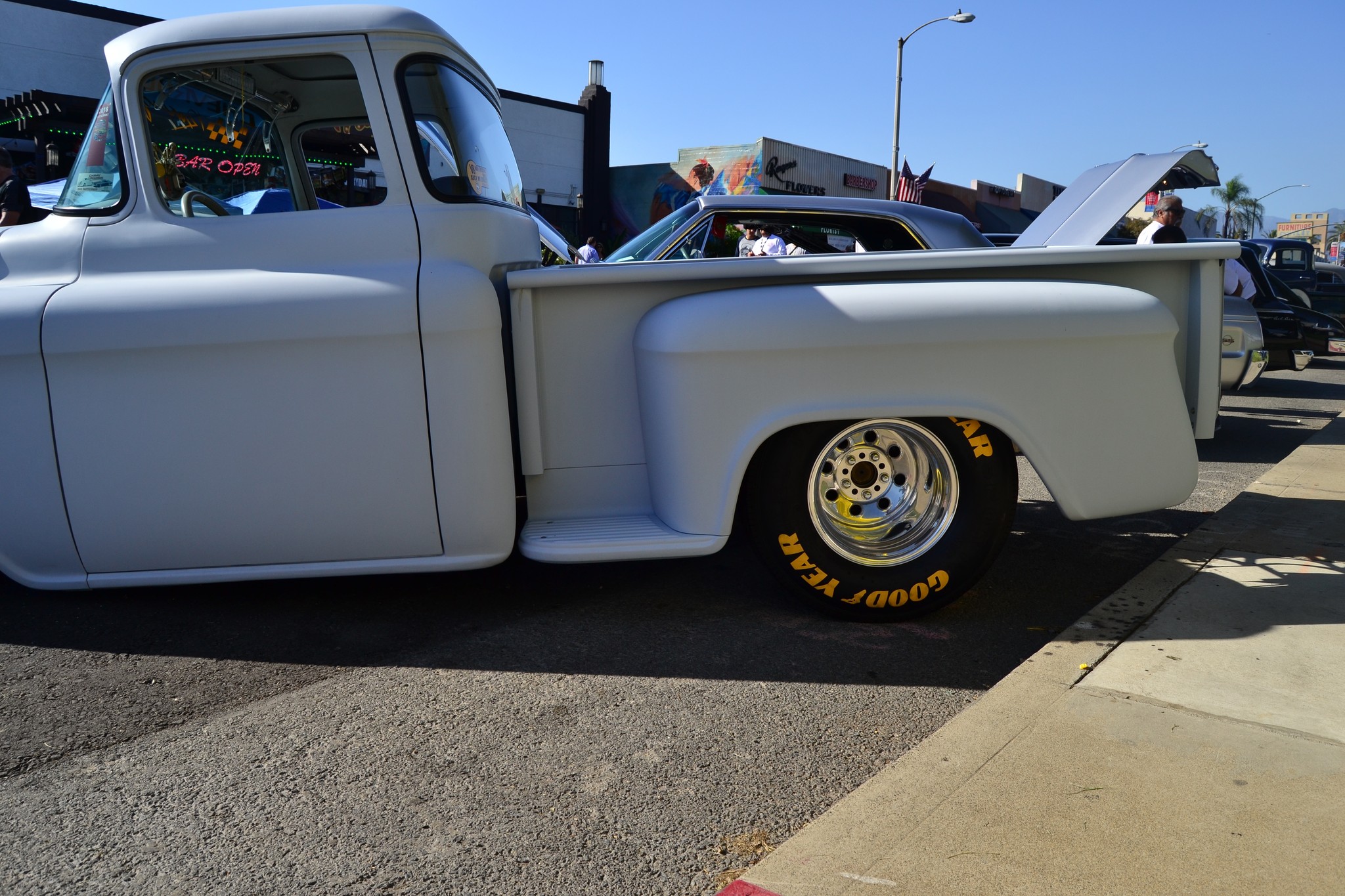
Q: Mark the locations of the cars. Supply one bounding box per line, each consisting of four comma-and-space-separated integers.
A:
526, 146, 1224, 269
1231, 237, 1345, 372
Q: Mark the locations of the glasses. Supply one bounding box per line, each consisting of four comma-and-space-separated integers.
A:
1163, 208, 1186, 215
597, 246, 602, 248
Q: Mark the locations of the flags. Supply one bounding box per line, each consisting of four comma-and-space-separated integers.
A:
896, 158, 924, 206
914, 163, 934, 192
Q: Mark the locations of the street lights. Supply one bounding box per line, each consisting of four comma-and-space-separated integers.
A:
1158, 141, 1209, 202
890, 9, 977, 202
1250, 184, 1312, 238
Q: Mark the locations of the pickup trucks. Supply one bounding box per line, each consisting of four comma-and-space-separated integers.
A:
0, 3, 1239, 619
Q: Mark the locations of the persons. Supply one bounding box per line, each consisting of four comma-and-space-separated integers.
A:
734, 225, 761, 257
1150, 223, 1257, 306
784, 242, 811, 255
0, 146, 34, 226
594, 243, 606, 261
682, 235, 705, 259
574, 236, 599, 265
746, 229, 787, 257
1136, 195, 1185, 246
